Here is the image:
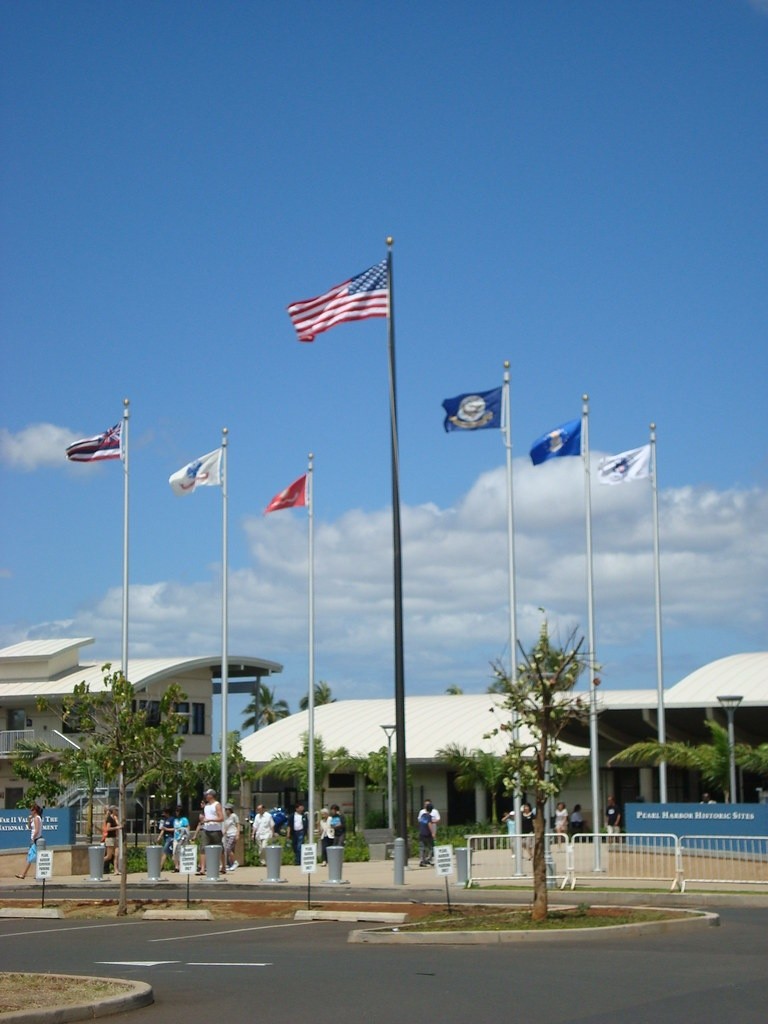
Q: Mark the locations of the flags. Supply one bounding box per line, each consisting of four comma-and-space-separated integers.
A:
264, 472, 309, 515
286, 257, 390, 342
441, 383, 506, 434
65, 419, 124, 462
528, 418, 584, 466
596, 442, 652, 487
169, 446, 223, 494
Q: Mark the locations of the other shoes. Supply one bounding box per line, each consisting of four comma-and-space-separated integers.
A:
419, 860, 434, 868
230, 862, 239, 870
14, 872, 25, 879
172, 868, 179, 874
115, 870, 121, 877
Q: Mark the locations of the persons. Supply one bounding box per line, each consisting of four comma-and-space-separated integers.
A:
99, 788, 621, 877
15, 804, 43, 879
698, 793, 717, 804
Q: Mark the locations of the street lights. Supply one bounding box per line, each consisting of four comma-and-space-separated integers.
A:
718, 694, 744, 803
379, 724, 397, 842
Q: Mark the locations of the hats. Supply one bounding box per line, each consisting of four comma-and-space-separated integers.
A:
509, 811, 515, 815
224, 804, 233, 811
203, 789, 217, 797
108, 805, 119, 813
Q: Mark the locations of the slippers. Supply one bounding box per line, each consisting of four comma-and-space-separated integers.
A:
218, 870, 226, 875
195, 872, 207, 875
